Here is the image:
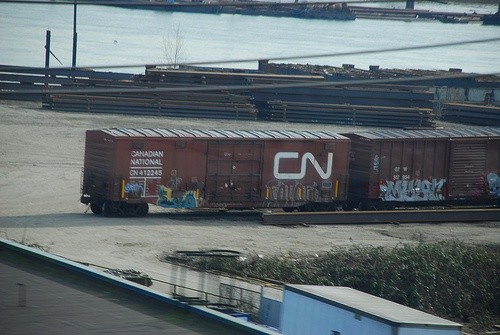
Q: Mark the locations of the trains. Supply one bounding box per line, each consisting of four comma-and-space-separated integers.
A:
79, 127, 500, 217
